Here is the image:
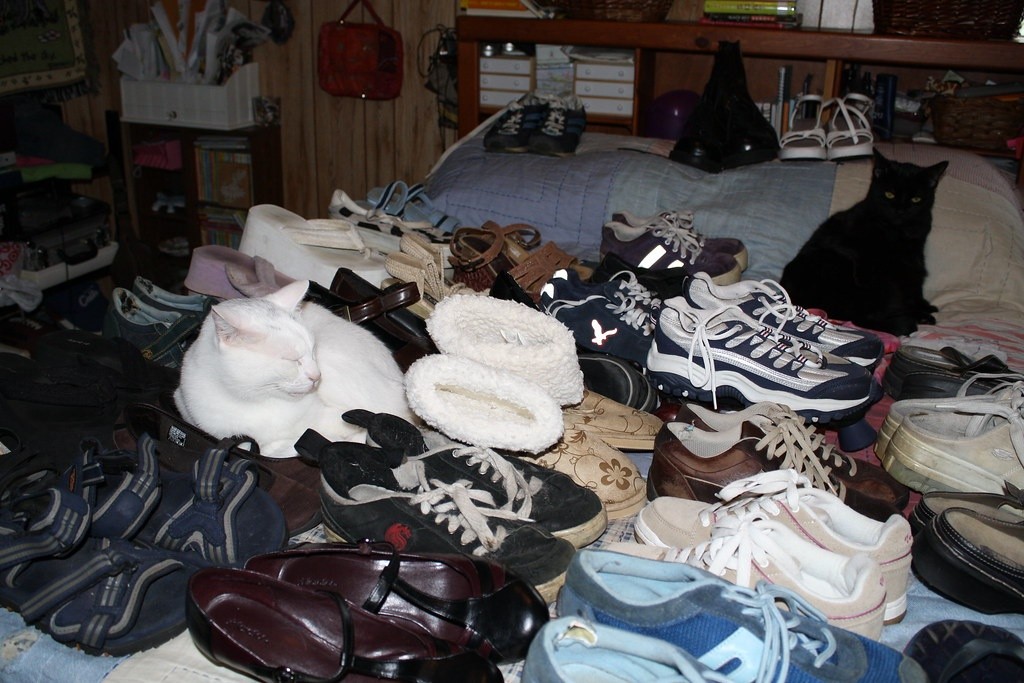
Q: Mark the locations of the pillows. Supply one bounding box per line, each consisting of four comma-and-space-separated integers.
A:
415, 131, 1024, 372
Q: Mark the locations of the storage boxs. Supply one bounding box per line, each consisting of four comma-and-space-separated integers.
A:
67, 241, 120, 279
537, 81, 573, 90
478, 73, 537, 93
477, 89, 530, 108
575, 59, 635, 82
535, 63, 575, 82
577, 95, 633, 121
18, 262, 67, 291
534, 88, 573, 100
536, 43, 585, 69
479, 56, 535, 76
573, 79, 635, 100
118, 61, 261, 131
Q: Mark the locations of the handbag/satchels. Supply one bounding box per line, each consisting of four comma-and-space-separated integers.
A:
318, 0, 404, 100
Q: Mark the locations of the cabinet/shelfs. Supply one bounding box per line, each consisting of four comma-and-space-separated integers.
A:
124, 124, 284, 274
455, 16, 1024, 194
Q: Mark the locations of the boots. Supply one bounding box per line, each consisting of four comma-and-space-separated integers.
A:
668, 40, 729, 173
725, 39, 782, 170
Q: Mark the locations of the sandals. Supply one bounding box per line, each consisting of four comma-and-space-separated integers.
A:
779, 95, 826, 161
827, 92, 874, 160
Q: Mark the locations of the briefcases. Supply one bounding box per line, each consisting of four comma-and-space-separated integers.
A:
0, 185, 114, 272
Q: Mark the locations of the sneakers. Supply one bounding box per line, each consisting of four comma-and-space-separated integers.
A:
0, 180, 1024, 683
532, 95, 586, 153
482, 94, 548, 153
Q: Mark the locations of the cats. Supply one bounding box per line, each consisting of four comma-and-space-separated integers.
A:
175, 279, 419, 458
779, 147, 949, 335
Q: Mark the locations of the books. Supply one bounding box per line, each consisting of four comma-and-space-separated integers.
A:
457, 0, 549, 18
700, 0, 803, 30
194, 148, 265, 209
201, 225, 242, 251
197, 204, 248, 230
755, 63, 815, 142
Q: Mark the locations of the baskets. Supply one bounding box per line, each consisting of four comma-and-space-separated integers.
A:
560, 0, 673, 24
872, 1, 1023, 42
931, 86, 1022, 150
